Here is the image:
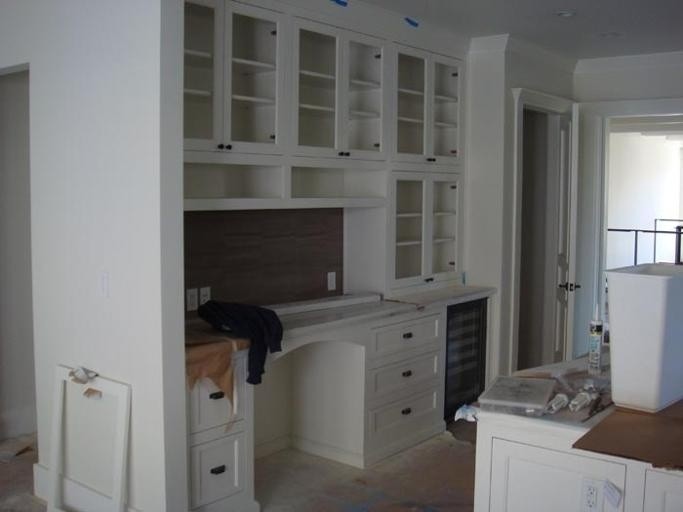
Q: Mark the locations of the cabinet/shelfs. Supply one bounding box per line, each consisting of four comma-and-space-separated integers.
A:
289, 302, 448, 471
183, 0, 285, 156
394, 171, 463, 291
292, 17, 387, 161
473, 357, 683, 511
185, 169, 385, 214
185, 337, 262, 512
395, 45, 464, 164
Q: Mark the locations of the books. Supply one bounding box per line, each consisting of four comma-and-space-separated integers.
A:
474, 374, 556, 407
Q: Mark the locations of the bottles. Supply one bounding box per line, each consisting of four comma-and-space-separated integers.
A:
588, 303, 602, 375
